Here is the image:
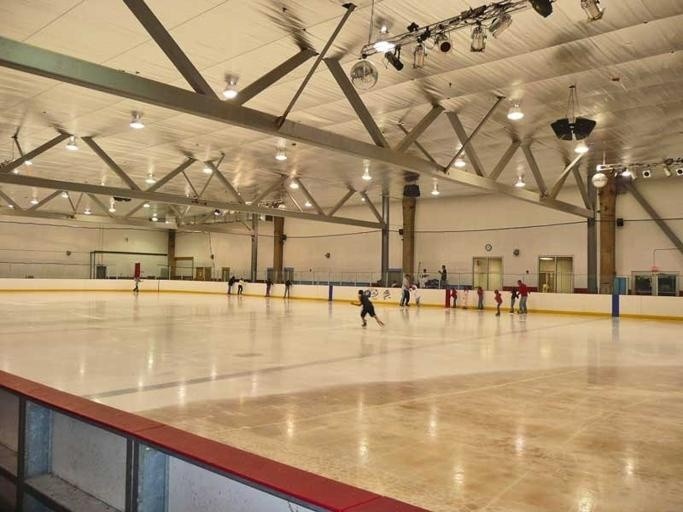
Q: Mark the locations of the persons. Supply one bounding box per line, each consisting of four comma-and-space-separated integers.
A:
132, 275, 143, 293
516, 280, 530, 313
282, 280, 292, 298
349, 289, 384, 328
262, 277, 273, 297
391, 265, 519, 317
226, 276, 235, 294
236, 277, 246, 296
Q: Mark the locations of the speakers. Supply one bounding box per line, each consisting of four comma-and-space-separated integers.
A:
551, 118, 573, 141
402, 184, 420, 196
617, 217, 623, 226
573, 117, 596, 140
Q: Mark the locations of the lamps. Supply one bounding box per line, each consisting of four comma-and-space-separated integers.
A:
507, 100, 523, 121
221, 72, 238, 98
346, 1, 558, 90
573, 139, 588, 156
65, 136, 77, 151
361, 166, 372, 181
430, 182, 439, 195
513, 175, 525, 187
129, 110, 144, 130
